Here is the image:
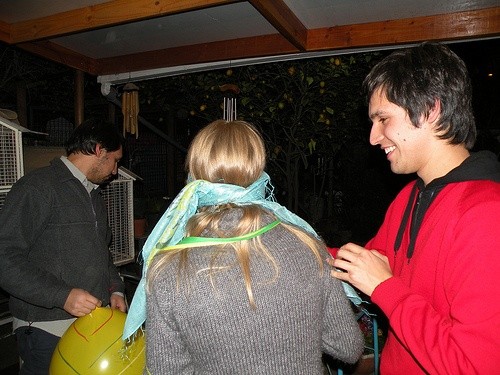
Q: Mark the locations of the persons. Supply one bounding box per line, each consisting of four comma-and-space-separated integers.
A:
326, 40, 499, 375
0, 118, 128, 375
144, 119, 365, 375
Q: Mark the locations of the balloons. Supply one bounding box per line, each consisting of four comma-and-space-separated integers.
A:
48, 305, 146, 375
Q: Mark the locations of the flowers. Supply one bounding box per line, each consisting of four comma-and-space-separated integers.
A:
355, 306, 383, 354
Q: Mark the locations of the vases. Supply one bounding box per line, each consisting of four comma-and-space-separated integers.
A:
135, 217, 147, 237
356, 354, 382, 374
